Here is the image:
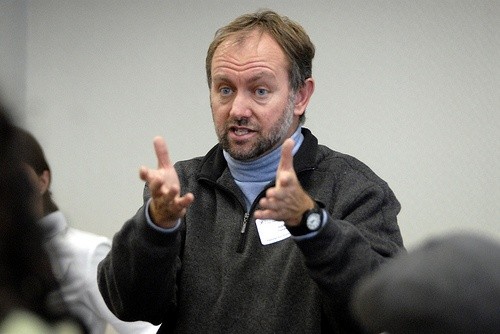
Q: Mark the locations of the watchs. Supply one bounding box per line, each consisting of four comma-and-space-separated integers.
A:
284, 196, 325, 236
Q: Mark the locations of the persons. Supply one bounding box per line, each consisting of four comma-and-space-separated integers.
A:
92, 9, 412, 333
12, 127, 164, 334
346, 228, 500, 334
0, 106, 90, 334
